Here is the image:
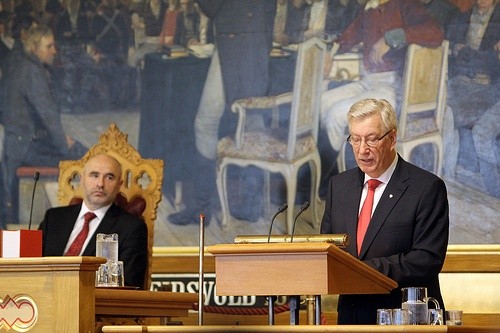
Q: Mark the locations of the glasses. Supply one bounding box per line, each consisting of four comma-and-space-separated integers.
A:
347, 130, 392, 146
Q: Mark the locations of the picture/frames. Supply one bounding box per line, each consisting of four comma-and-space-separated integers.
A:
0, 0, 500, 272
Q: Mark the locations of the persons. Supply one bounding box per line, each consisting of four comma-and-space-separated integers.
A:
320, 97, 449, 326
37, 153, 149, 291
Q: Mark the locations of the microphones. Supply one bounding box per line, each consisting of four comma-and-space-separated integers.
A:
268, 203, 288, 243
291, 202, 310, 243
29, 172, 40, 229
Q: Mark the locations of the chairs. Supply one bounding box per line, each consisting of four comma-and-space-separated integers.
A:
57, 123, 165, 291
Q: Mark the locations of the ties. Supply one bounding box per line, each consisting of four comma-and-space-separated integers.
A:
357, 180, 382, 256
64, 212, 96, 256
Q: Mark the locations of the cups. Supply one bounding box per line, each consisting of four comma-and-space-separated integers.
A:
401, 287, 440, 326
375, 307, 464, 326
96, 261, 125, 287
95, 234, 119, 286
